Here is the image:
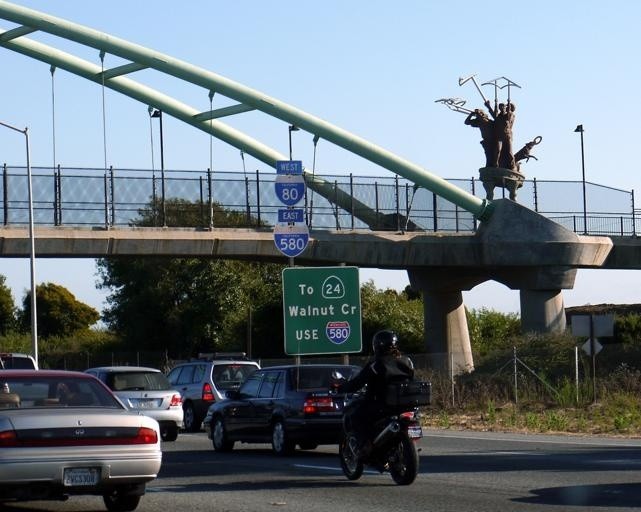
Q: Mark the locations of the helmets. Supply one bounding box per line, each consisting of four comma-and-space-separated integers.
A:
370, 329, 397, 353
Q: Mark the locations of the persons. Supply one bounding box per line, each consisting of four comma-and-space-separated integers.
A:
466, 109, 498, 167
493, 103, 516, 170
333, 330, 415, 457
485, 99, 516, 129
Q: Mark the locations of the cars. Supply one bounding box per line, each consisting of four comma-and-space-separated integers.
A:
0, 352, 184, 512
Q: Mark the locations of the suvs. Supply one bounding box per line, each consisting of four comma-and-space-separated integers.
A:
202, 361, 367, 461
163, 352, 264, 437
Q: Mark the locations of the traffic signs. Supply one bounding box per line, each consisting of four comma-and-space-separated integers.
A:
275, 174, 305, 205
272, 224, 309, 258
275, 160, 301, 176
280, 266, 362, 355
277, 209, 302, 222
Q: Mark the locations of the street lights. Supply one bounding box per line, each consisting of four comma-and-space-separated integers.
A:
288, 126, 299, 161
572, 123, 589, 234
152, 108, 166, 228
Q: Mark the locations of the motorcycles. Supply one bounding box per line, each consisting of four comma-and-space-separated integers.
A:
329, 370, 434, 487
